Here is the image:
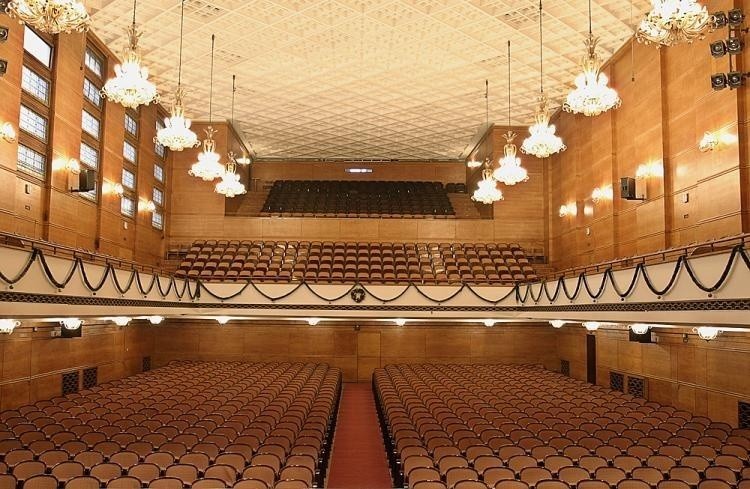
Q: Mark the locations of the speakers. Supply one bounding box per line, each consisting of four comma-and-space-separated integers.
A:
620, 177, 635, 199
79, 170, 95, 190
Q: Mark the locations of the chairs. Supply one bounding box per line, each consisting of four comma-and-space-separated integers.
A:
258, 179, 467, 219
368, 362, 746, 487
2, 362, 344, 489
174, 238, 542, 286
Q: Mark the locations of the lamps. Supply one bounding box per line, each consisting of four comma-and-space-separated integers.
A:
139, 198, 158, 215
710, 7, 750, 92
520, 0, 566, 158
1, 120, 18, 147
698, 131, 719, 153
470, 80, 504, 206
634, 0, 715, 49
189, 33, 228, 184
3, 2, 96, 38
492, 42, 529, 186
55, 156, 84, 177
546, 317, 725, 342
155, 3, 203, 155
561, 0, 621, 118
213, 73, 246, 200
0, 315, 165, 335
96, 0, 162, 111
107, 182, 123, 196
